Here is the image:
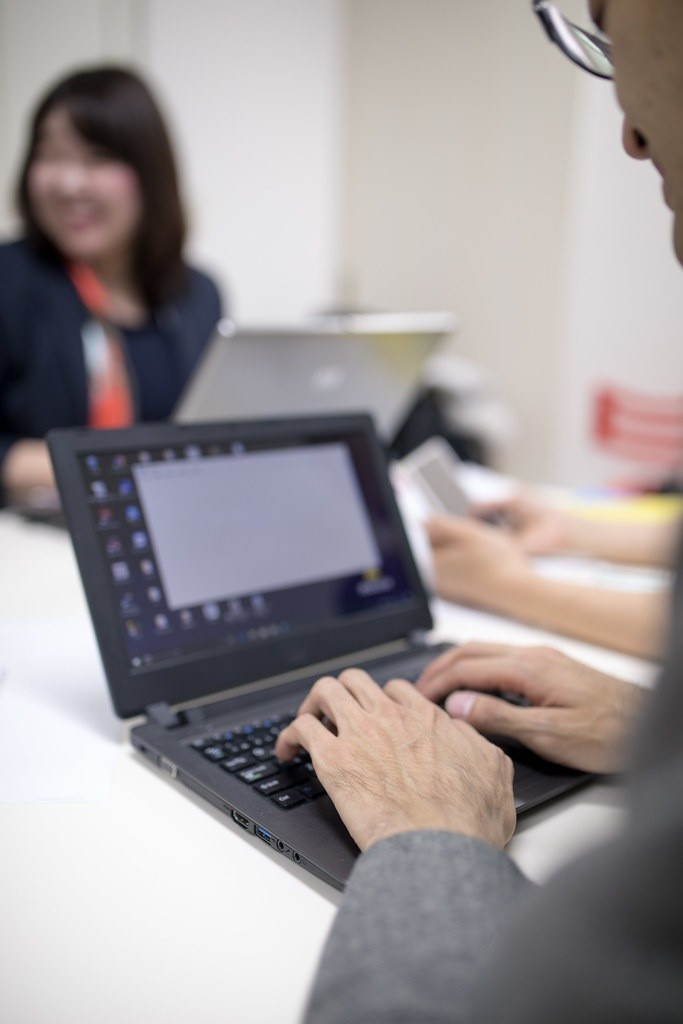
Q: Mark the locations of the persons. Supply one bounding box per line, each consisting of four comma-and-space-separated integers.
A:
425, 492, 682, 663
276, 3, 683, 1024
0, 66, 222, 518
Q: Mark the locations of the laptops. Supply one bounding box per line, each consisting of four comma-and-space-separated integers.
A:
46, 411, 601, 889
171, 314, 457, 445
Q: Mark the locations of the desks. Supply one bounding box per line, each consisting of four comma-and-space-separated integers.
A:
0, 478, 683, 1024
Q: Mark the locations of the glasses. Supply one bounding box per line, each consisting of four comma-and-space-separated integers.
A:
531, 3, 618, 87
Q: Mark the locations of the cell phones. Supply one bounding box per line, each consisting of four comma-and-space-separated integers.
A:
412, 454, 475, 522
